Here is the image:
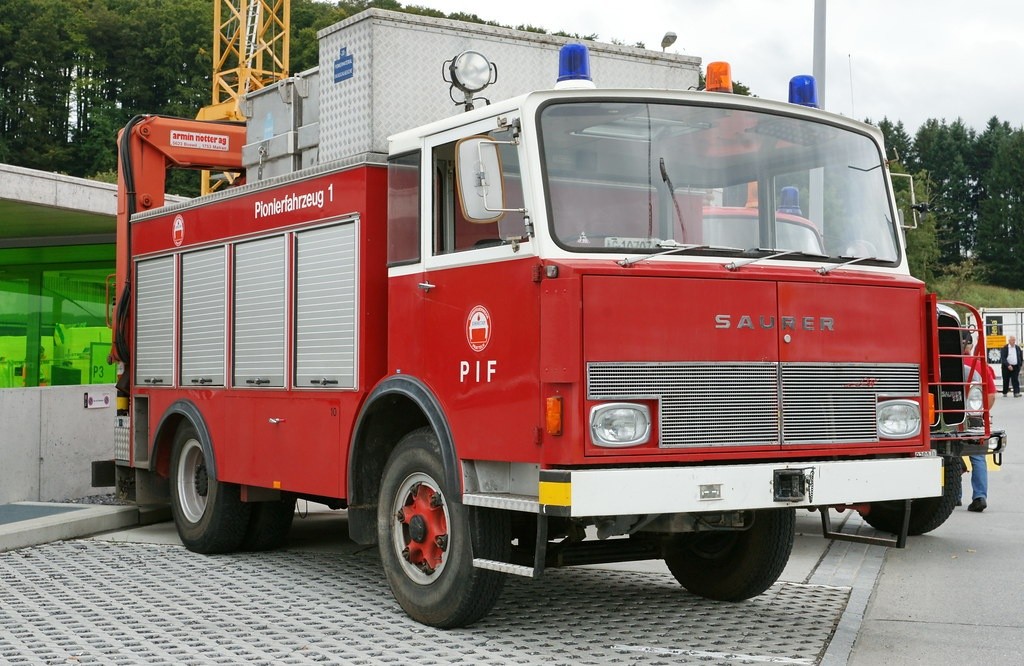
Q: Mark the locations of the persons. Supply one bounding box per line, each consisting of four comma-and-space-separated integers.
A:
1001, 336, 1024, 397
955, 329, 987, 511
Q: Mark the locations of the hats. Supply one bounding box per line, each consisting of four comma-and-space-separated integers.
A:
962, 330, 972, 344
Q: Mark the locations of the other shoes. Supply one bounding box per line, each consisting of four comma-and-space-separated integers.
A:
1004, 393, 1007, 397
968, 497, 986, 512
1014, 394, 1022, 397
956, 499, 962, 506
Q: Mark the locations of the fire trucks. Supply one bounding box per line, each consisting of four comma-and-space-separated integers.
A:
87, 1, 1008, 630
